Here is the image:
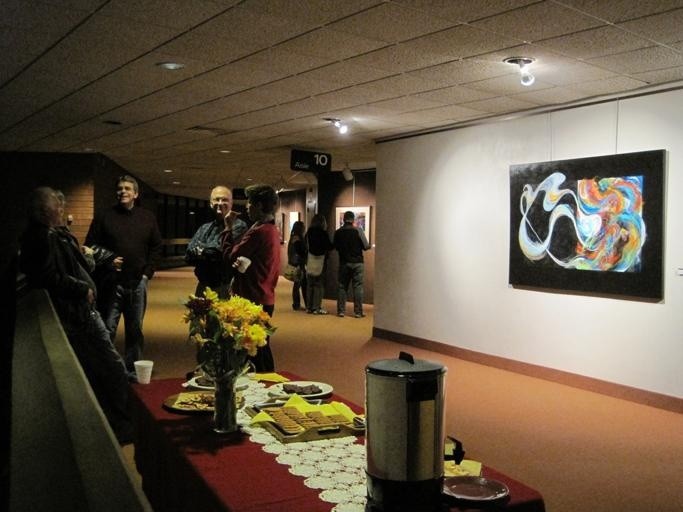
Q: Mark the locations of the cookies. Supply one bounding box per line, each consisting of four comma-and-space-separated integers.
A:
264, 405, 351, 435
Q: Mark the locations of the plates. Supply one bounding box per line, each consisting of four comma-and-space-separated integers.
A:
162, 390, 246, 414
441, 475, 510, 504
267, 379, 335, 399
188, 375, 250, 390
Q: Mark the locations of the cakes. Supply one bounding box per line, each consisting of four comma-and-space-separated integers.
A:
353, 417, 365, 428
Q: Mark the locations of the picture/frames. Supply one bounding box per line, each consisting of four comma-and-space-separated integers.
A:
334, 205, 371, 248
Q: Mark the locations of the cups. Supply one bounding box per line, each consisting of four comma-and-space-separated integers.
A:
133, 359, 153, 384
236, 256, 251, 274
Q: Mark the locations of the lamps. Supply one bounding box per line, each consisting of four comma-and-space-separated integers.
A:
340, 159, 355, 182
322, 118, 348, 134
502, 57, 536, 87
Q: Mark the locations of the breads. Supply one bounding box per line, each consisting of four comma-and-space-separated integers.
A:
283, 383, 322, 395
195, 376, 213, 386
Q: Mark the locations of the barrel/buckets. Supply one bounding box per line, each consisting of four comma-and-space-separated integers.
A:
363, 352, 447, 512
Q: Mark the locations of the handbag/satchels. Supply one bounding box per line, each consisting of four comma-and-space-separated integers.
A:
306, 252, 325, 277
283, 263, 304, 283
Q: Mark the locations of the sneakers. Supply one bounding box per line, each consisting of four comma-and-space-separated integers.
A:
294, 305, 328, 314
356, 313, 365, 318
338, 313, 344, 317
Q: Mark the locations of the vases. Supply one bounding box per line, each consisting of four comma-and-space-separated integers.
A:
211, 346, 238, 434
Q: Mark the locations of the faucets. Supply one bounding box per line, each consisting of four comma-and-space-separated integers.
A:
445, 434, 465, 466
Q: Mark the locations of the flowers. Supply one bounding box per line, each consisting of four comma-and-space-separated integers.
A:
177, 278, 278, 430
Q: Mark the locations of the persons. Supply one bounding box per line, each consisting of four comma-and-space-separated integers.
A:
219, 183, 281, 372
334, 211, 370, 318
20, 175, 162, 431
288, 221, 308, 310
305, 213, 333, 314
185, 184, 249, 364
